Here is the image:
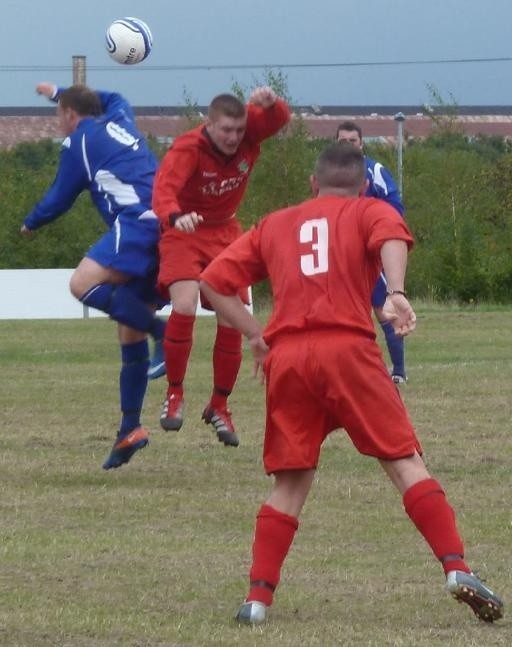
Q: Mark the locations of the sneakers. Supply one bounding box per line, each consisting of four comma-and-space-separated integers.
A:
147, 339, 166, 379
102, 422, 149, 469
160, 395, 185, 430
235, 598, 266, 625
446, 571, 503, 623
202, 403, 239, 447
391, 375, 408, 384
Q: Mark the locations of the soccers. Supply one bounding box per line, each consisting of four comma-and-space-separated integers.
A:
104, 17, 152, 64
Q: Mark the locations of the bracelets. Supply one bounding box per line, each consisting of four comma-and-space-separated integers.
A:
382, 287, 408, 302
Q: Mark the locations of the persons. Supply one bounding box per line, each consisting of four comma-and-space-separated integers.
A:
152, 85, 287, 447
22, 77, 161, 471
333, 124, 409, 386
199, 141, 505, 624
